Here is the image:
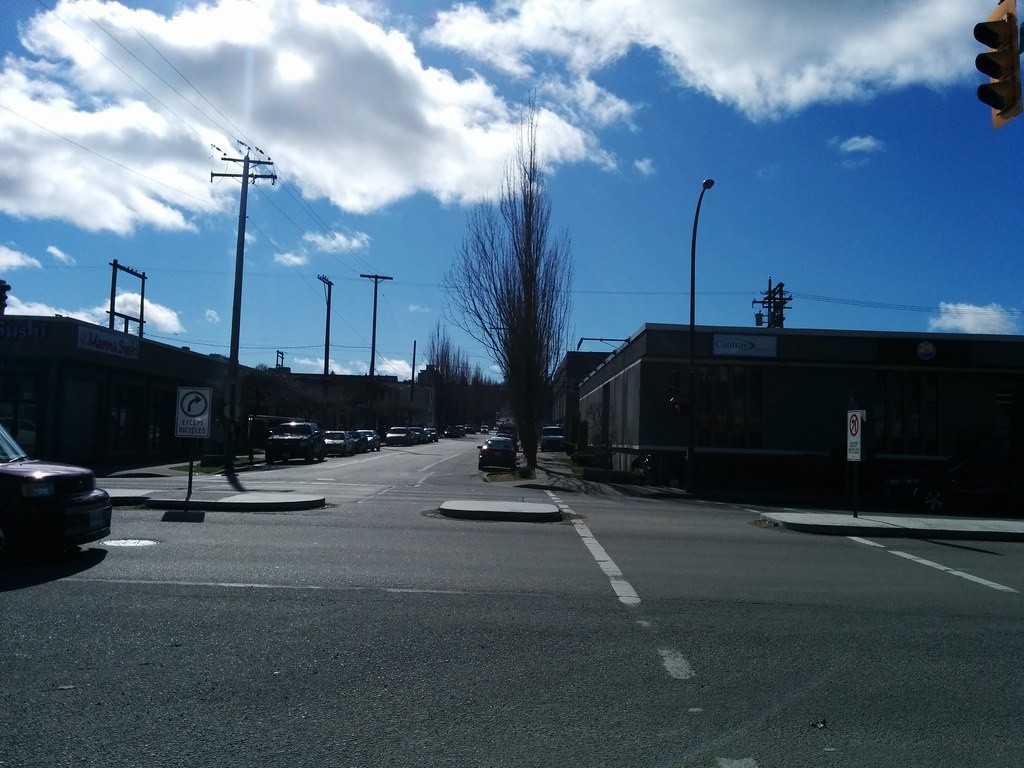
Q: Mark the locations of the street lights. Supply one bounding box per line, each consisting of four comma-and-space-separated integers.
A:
684, 179, 717, 494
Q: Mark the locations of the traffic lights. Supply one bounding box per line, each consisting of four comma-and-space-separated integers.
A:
971, 0, 1023, 131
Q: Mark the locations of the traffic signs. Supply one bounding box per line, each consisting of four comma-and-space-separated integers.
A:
174, 386, 213, 440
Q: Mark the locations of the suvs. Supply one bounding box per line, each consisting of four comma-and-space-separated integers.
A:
263, 421, 326, 464
0, 425, 114, 555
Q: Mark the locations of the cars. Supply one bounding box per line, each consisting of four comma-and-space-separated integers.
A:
476, 436, 517, 471
489, 421, 519, 450
324, 430, 355, 457
541, 425, 566, 451
444, 423, 475, 439
355, 429, 381, 452
480, 425, 489, 435
385, 426, 439, 446
